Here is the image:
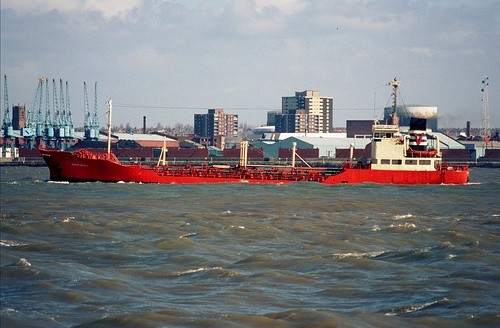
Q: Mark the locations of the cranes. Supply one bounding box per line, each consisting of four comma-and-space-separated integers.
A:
0, 71, 102, 167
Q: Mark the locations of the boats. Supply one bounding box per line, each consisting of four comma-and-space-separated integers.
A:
33, 76, 471, 187
